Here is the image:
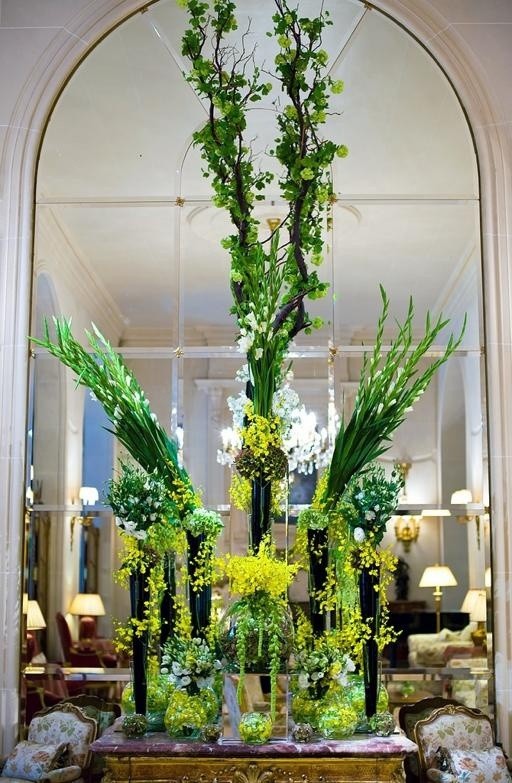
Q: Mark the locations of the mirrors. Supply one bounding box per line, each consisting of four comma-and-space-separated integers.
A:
1, 2, 508, 736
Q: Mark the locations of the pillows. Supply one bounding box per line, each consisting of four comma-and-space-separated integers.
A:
2, 741, 72, 781
440, 745, 510, 782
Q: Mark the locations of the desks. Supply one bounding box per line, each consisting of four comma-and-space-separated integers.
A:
85, 723, 421, 783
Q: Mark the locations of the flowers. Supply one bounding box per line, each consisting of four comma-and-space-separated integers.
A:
25, 0, 479, 741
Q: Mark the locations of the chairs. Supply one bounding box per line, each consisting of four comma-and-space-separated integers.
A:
418, 708, 511, 783
0, 704, 99, 783
